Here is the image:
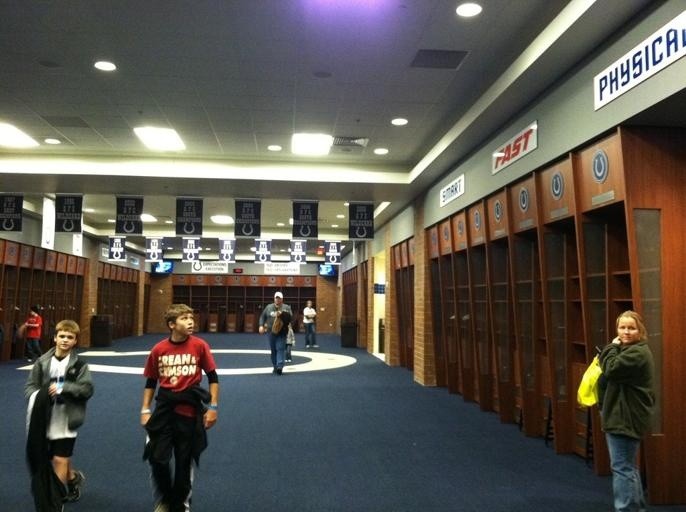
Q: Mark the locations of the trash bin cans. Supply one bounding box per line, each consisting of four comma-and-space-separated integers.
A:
340, 316, 358, 348
90, 314, 113, 347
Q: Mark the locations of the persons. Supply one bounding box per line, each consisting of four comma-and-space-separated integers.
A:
17, 306, 43, 363
23, 320, 94, 511
284, 321, 296, 363
595, 310, 657, 511
140, 303, 220, 512
303, 299, 321, 349
258, 292, 293, 374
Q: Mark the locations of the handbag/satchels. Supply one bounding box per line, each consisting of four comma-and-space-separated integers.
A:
577, 354, 605, 408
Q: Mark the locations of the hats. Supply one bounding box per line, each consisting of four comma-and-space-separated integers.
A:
30, 306, 38, 313
274, 292, 284, 299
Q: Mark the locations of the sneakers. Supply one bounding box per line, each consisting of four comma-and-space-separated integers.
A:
67, 470, 85, 503
285, 359, 292, 363
273, 367, 283, 376
306, 344, 319, 348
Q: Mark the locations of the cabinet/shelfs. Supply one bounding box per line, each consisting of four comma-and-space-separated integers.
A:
394, 264, 414, 372
428, 200, 650, 508
95, 277, 150, 339
1, 264, 84, 368
339, 281, 359, 347
171, 284, 317, 335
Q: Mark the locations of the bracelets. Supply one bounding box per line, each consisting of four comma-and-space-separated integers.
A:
57, 381, 63, 394
141, 407, 152, 414
200, 401, 219, 411
